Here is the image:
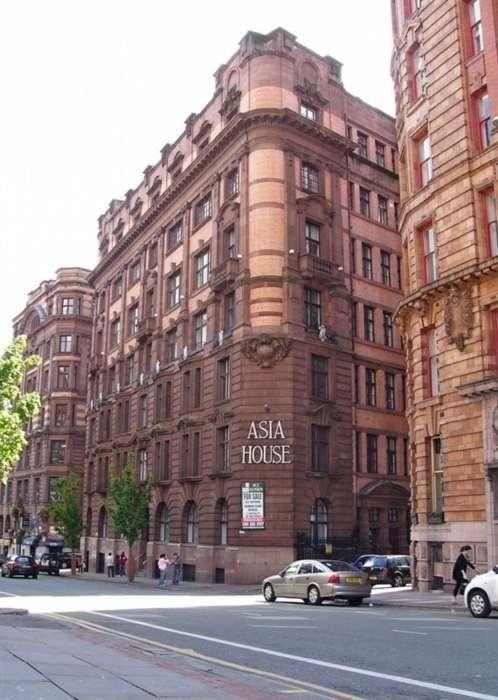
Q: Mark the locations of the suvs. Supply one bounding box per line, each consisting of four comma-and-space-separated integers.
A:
35, 552, 60, 577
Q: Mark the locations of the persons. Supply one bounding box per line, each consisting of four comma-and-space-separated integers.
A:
449, 545, 480, 605
156, 553, 169, 588
104, 553, 116, 578
169, 552, 182, 586
118, 551, 128, 576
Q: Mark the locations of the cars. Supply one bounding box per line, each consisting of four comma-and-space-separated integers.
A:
361, 554, 411, 587
0, 555, 38, 578
58, 551, 81, 568
349, 554, 368, 569
463, 563, 497, 617
259, 556, 371, 609
